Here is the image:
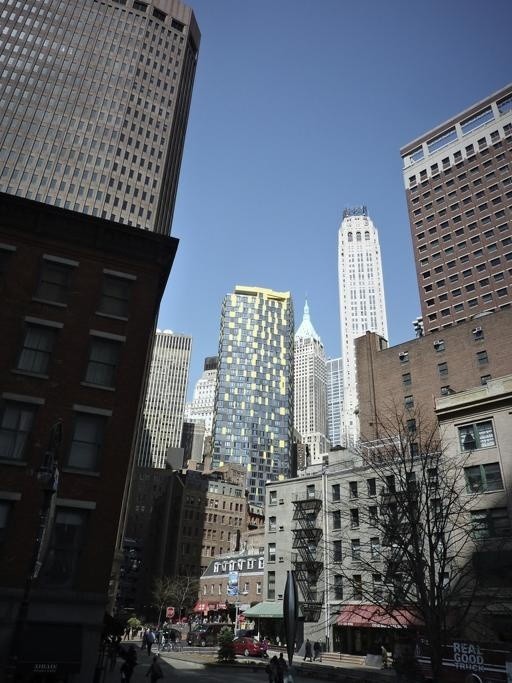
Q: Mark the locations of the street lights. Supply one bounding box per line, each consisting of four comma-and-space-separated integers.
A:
330, 444, 443, 682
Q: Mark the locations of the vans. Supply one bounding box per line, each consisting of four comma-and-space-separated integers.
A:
186, 622, 238, 647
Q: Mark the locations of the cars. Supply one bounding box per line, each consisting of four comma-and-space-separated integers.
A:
232, 636, 267, 656
153, 628, 182, 643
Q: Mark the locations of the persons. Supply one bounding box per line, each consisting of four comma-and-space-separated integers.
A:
380, 644, 387, 664
303, 638, 323, 662
140, 628, 155, 656
260, 635, 270, 659
124, 627, 131, 640
145, 656, 163, 683
265, 653, 288, 683
